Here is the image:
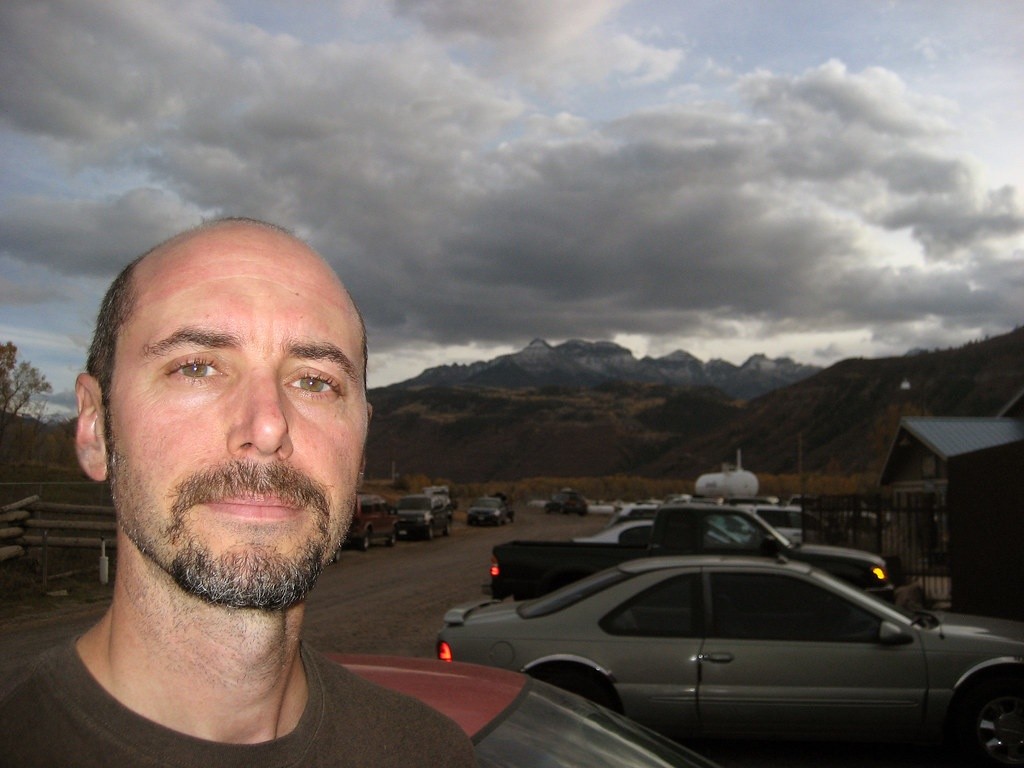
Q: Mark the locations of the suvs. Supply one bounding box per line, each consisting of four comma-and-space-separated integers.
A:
339, 493, 399, 551
392, 494, 454, 541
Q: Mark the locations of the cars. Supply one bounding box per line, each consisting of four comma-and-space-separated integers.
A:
570, 489, 891, 554
327, 650, 725, 768
541, 487, 587, 516
466, 494, 515, 527
431, 555, 1024, 768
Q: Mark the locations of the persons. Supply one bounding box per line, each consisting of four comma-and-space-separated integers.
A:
0, 218, 479, 768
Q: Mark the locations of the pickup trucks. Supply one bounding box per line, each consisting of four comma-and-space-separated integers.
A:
489, 501, 899, 615
